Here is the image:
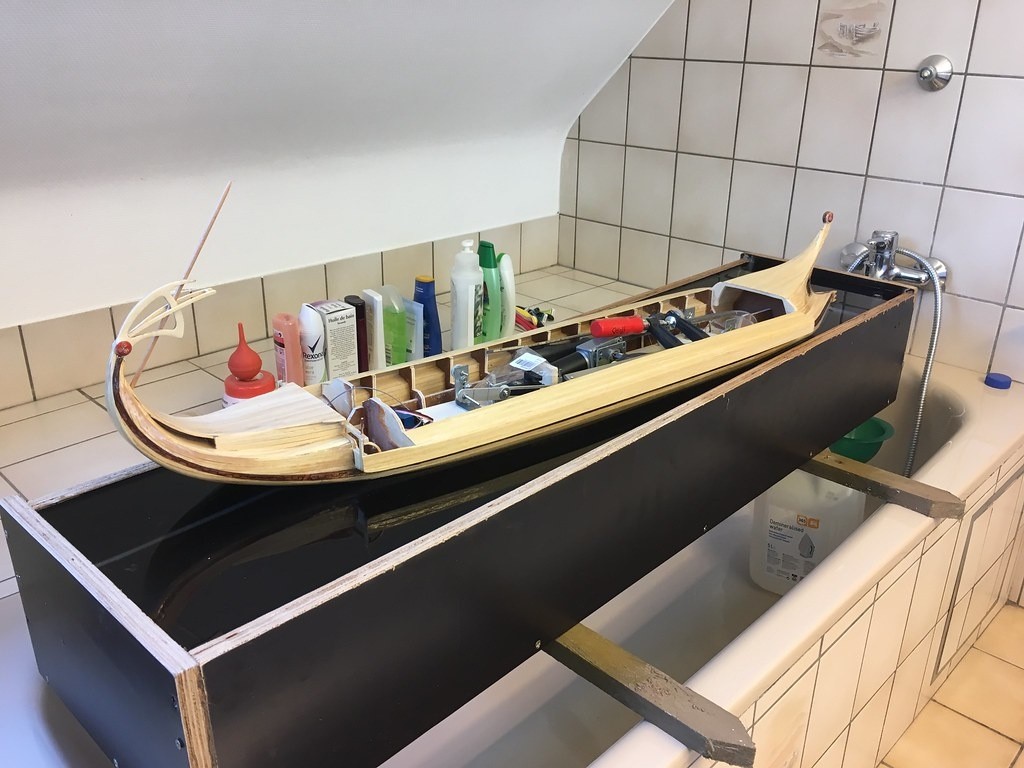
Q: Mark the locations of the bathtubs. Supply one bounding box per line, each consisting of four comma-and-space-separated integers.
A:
0, 263, 1024, 768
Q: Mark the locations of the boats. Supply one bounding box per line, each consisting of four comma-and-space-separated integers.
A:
106, 211, 839, 489
145, 487, 620, 624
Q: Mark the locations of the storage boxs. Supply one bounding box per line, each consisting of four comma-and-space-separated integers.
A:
315, 300, 358, 381
0, 251, 918, 768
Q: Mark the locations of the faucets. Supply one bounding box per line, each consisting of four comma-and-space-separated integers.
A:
865, 229, 901, 292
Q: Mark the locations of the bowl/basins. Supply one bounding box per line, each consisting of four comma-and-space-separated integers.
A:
829, 416, 894, 463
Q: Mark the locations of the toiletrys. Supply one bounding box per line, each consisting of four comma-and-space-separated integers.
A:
342, 275, 443, 373
450, 240, 516, 351
271, 313, 304, 389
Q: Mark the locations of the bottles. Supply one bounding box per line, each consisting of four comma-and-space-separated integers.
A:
748, 470, 866, 596
